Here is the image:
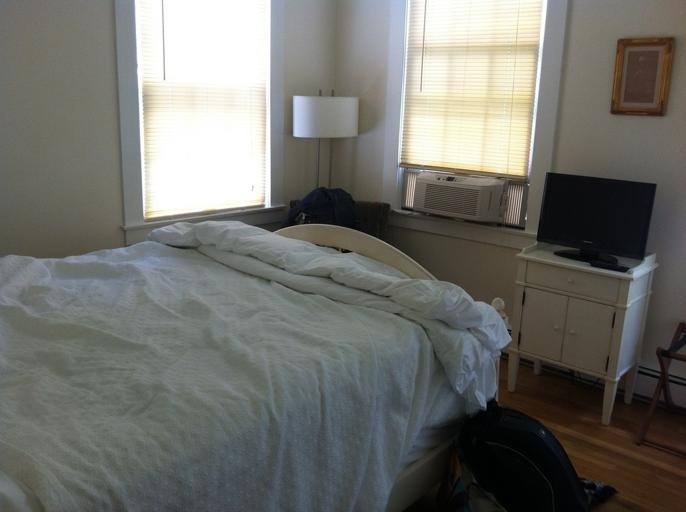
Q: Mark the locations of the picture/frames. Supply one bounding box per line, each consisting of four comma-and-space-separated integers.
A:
609, 33, 677, 118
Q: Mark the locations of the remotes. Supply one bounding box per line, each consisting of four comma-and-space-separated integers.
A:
590, 259, 631, 273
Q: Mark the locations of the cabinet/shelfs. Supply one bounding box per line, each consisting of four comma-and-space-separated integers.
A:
506, 241, 659, 426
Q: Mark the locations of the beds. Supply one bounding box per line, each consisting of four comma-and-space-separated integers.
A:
1, 223, 510, 512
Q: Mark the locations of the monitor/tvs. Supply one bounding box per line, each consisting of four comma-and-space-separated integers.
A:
536, 172, 658, 261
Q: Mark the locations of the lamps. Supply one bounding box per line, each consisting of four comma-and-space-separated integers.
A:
290, 94, 361, 194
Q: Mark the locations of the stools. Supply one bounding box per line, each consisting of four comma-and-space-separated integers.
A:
633, 320, 686, 462
